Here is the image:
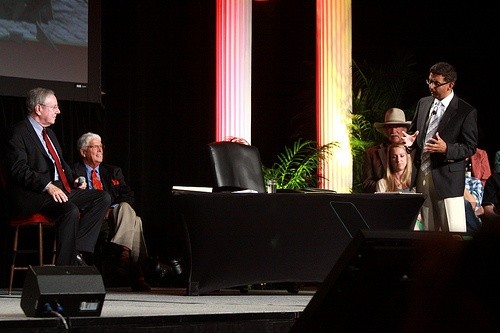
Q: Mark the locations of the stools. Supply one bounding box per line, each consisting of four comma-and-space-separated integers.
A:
8, 211, 57, 296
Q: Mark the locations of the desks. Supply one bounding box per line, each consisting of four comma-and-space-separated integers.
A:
161, 192, 427, 294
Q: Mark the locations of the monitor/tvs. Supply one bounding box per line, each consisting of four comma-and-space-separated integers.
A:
288, 230, 474, 333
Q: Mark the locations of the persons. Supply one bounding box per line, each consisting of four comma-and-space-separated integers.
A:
362, 62, 500, 232
3, 88, 110, 266
72, 131, 157, 292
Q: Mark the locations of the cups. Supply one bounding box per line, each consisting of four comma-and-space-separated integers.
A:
267, 180, 276, 193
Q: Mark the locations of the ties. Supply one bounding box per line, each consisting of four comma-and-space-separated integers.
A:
419, 102, 442, 176
92, 169, 103, 191
43, 127, 71, 193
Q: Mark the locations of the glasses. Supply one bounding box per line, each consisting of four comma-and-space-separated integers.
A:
85, 145, 106, 150
38, 103, 59, 111
426, 78, 450, 89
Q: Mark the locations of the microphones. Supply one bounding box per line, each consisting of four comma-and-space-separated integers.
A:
76, 176, 85, 187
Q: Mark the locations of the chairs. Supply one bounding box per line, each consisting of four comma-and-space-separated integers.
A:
208, 141, 299, 293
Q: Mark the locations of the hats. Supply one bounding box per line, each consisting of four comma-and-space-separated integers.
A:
374, 108, 412, 134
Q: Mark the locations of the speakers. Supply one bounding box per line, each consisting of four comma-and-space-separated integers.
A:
21, 265, 106, 317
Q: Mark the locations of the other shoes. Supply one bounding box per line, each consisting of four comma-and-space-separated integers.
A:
132, 280, 151, 291
115, 247, 134, 276
71, 251, 87, 265
